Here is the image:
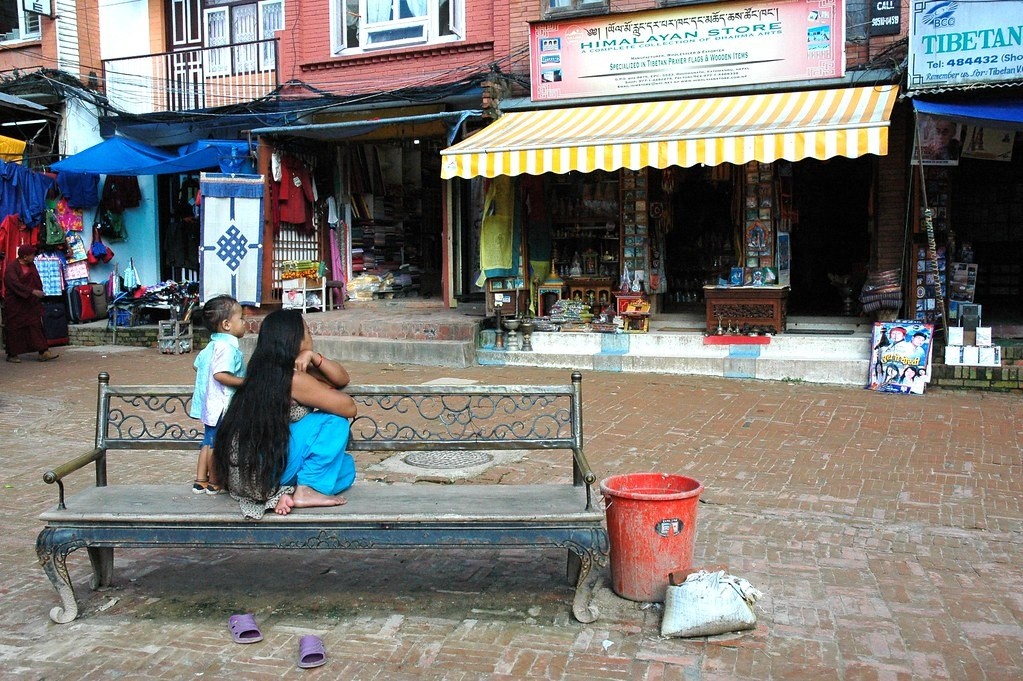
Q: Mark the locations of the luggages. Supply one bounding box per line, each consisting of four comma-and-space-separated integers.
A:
67, 279, 107, 323
41, 299, 70, 347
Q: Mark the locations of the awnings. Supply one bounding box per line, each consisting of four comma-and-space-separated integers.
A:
440, 85, 899, 180
48, 136, 258, 176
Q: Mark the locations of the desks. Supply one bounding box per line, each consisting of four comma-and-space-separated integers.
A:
702, 285, 790, 333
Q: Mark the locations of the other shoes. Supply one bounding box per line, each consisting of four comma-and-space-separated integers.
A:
6, 354, 21, 362
206, 484, 229, 494
192, 481, 210, 494
38, 350, 59, 362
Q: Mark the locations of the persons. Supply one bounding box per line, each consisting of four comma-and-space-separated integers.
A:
189, 296, 246, 495
212, 310, 357, 516
4, 245, 59, 363
929, 121, 960, 160
172, 206, 200, 281
874, 328, 926, 395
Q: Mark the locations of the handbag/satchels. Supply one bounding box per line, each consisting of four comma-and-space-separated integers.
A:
45, 189, 127, 285
34, 252, 64, 296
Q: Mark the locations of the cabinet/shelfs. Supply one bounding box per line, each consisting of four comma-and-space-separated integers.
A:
353, 144, 442, 294
282, 275, 326, 312
620, 167, 649, 293
742, 161, 773, 269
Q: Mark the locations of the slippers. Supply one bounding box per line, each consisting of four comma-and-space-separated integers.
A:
228, 614, 263, 643
298, 634, 327, 668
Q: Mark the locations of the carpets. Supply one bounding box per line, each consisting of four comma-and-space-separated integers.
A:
657, 326, 706, 333
704, 336, 771, 345
784, 328, 855, 334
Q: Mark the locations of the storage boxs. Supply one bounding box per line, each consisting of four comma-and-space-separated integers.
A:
917, 243, 1002, 368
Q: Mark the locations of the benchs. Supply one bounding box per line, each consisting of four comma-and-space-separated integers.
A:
36, 370, 611, 624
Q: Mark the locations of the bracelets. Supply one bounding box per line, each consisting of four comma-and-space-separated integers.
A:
313, 353, 323, 368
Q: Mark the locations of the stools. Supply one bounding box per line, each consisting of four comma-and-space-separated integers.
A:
325, 280, 345, 311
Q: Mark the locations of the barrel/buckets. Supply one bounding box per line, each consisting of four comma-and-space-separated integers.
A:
599, 473, 704, 602
